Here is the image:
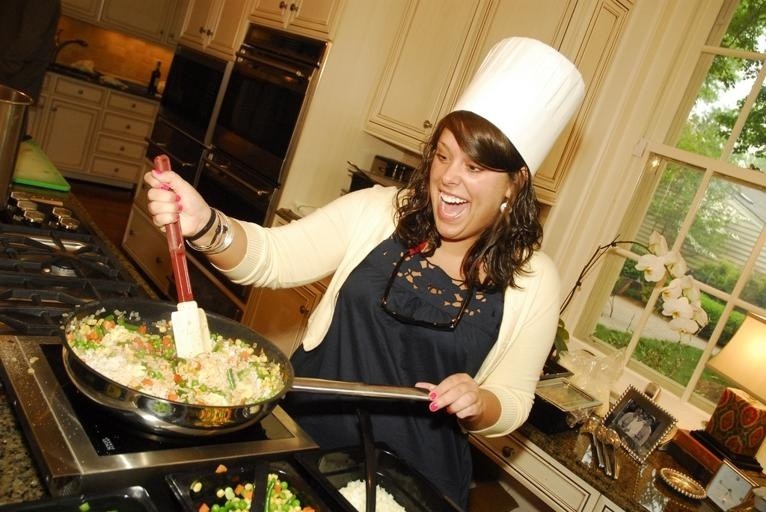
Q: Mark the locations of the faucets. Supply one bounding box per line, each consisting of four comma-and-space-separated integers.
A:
52, 38, 89, 60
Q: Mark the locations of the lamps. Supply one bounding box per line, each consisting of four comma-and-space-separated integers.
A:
689, 311, 766, 472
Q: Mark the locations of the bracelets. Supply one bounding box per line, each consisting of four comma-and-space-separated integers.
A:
186, 207, 235, 255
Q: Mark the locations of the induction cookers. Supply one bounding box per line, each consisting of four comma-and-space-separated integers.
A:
0, 336, 322, 501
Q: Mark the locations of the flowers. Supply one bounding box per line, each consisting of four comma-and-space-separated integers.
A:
538, 231, 710, 376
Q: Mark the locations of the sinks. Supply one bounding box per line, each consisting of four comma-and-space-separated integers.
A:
53, 61, 99, 82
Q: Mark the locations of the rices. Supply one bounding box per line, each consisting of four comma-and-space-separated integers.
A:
339, 478, 406, 512
67, 308, 286, 408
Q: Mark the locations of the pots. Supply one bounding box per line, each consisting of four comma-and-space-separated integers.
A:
1, 81, 34, 219
59, 297, 438, 443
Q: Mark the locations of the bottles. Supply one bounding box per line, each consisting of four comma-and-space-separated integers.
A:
146, 61, 163, 100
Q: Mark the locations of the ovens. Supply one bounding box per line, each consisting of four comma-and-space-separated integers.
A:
122, 23, 328, 309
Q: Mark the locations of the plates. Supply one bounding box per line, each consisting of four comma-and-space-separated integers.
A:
658, 466, 705, 501
651, 478, 700, 512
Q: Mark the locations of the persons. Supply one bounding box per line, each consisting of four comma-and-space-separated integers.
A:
141, 110, 564, 509
616, 406, 656, 446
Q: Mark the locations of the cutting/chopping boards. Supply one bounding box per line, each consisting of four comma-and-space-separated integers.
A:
10, 136, 72, 195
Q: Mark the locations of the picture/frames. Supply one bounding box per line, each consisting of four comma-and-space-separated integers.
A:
598, 382, 678, 464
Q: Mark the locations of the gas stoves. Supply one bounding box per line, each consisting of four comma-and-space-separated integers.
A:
0, 224, 152, 336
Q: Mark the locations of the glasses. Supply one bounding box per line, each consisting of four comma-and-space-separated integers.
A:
380, 251, 475, 332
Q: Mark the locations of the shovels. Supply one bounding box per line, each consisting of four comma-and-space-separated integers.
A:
154, 151, 213, 362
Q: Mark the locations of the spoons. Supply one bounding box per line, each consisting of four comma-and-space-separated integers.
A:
586, 417, 622, 480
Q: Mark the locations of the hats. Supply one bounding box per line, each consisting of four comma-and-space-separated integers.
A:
449, 36, 587, 178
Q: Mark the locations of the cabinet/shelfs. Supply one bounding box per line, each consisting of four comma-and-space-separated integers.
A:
365, 0, 629, 191
175, 0, 251, 62
245, 283, 322, 359
88, 92, 158, 184
466, 429, 600, 511
248, 1, 344, 41
58, 1, 188, 49
27, 73, 107, 173
123, 160, 171, 292
